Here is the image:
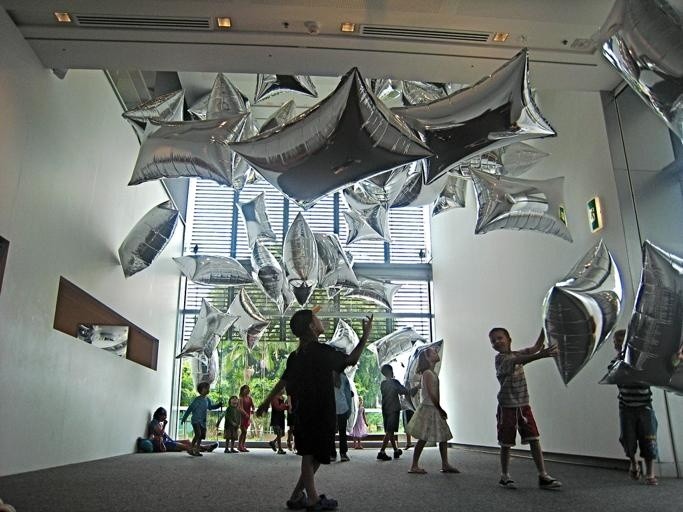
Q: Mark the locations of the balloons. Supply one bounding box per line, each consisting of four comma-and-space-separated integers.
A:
281, 210, 319, 305
172, 255, 255, 286
312, 231, 359, 291
186, 92, 209, 123
259, 97, 298, 134
251, 72, 318, 103
121, 87, 187, 146
205, 72, 245, 123
388, 45, 558, 186
433, 176, 465, 217
445, 142, 552, 182
367, 326, 429, 369
235, 192, 276, 249
597, 239, 683, 399
439, 82, 471, 97
330, 316, 364, 381
383, 159, 424, 210
467, 166, 576, 245
588, 1, 683, 144
542, 235, 623, 386
361, 75, 403, 107
400, 338, 445, 414
340, 180, 389, 211
400, 80, 448, 107
342, 274, 402, 312
184, 348, 219, 393
343, 249, 354, 268
126, 113, 250, 189
225, 64, 437, 209
340, 201, 394, 245
119, 199, 180, 279
174, 296, 241, 371
226, 285, 271, 353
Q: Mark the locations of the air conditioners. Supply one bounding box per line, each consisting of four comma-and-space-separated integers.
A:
70, 13, 213, 31
358, 24, 494, 45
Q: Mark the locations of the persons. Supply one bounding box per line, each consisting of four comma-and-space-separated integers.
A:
268, 388, 289, 454
329, 372, 351, 462
405, 348, 460, 477
489, 328, 563, 492
223, 396, 241, 454
350, 396, 369, 450
149, 407, 218, 452
608, 329, 660, 487
376, 364, 421, 462
287, 393, 299, 452
180, 382, 225, 456
237, 383, 255, 451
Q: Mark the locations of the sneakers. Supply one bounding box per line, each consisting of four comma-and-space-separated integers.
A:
287, 443, 293, 451
277, 449, 286, 454
352, 444, 357, 448
377, 453, 392, 460
340, 454, 350, 462
230, 447, 238, 453
268, 441, 277, 451
239, 446, 244, 451
187, 449, 195, 455
539, 474, 563, 489
355, 445, 363, 449
207, 442, 219, 452
330, 454, 337, 461
224, 449, 231, 452
394, 450, 402, 458
645, 474, 660, 486
628, 462, 642, 481
193, 451, 202, 456
499, 479, 519, 488
200, 446, 207, 452
243, 448, 249, 452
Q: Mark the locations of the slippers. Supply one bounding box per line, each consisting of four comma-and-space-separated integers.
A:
287, 492, 308, 508
308, 494, 337, 510
403, 444, 414, 450
407, 468, 427, 473
439, 469, 462, 472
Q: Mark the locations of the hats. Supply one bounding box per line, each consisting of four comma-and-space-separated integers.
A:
289, 306, 320, 338
